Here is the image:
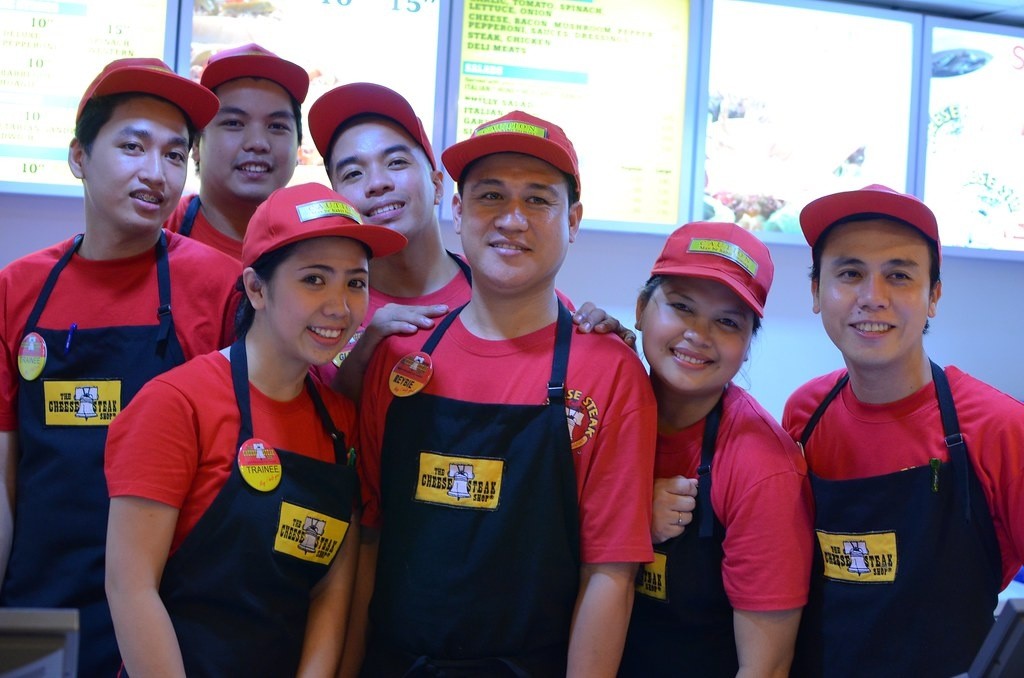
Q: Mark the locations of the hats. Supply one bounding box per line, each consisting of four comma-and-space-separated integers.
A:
76, 58, 221, 134
798, 183, 943, 268
241, 182, 410, 276
307, 82, 437, 172
200, 42, 310, 105
651, 220, 776, 319
441, 110, 582, 201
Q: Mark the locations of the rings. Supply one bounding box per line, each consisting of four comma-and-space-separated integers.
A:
677, 510, 682, 523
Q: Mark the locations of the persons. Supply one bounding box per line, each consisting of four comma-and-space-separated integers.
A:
631, 223, 814, 678
103, 183, 372, 678
334, 108, 656, 678
0, 53, 250, 676
778, 185, 1024, 678
156, 43, 307, 267
306, 77, 635, 405
